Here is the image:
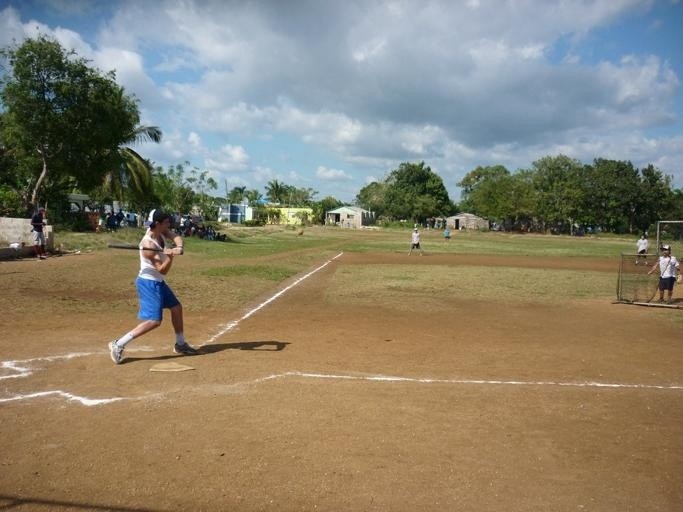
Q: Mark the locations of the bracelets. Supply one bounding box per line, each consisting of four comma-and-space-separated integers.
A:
175, 244, 183, 248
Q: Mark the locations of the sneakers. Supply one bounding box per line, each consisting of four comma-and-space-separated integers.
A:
174, 342, 197, 355
658, 299, 671, 303
109, 340, 123, 364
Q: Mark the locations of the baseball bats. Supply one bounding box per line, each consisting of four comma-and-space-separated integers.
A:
106, 243, 183, 255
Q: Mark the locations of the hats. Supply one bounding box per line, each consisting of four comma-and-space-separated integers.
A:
143, 208, 168, 228
660, 245, 671, 251
414, 228, 418, 231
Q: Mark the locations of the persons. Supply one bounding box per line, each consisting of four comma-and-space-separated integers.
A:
105, 208, 227, 242
646, 244, 682, 304
442, 225, 451, 241
407, 226, 423, 257
429, 215, 446, 230
30, 206, 50, 259
106, 208, 198, 365
634, 234, 649, 266
